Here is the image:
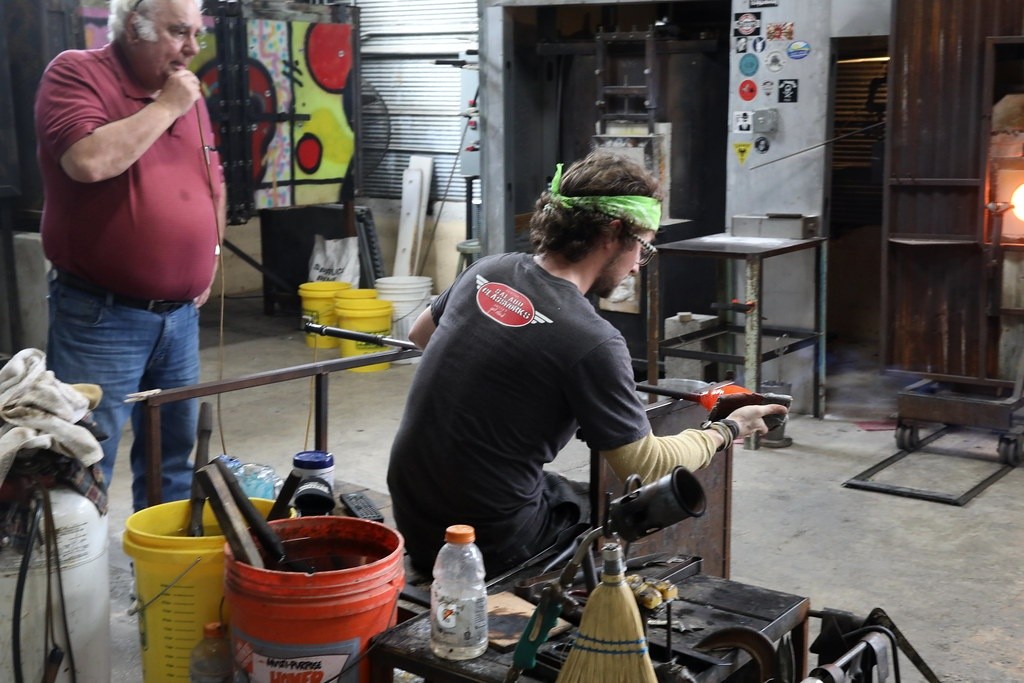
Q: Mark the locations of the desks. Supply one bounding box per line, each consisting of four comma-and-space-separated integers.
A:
369, 555, 811, 683
646, 233, 828, 451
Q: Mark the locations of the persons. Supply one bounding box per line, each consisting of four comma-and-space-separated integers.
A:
35, 0, 226, 604
737, 113, 751, 132
385, 141, 789, 589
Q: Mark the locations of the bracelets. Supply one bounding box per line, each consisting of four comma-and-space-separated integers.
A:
699, 419, 739, 452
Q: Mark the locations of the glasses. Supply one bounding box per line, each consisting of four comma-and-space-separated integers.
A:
629, 232, 657, 266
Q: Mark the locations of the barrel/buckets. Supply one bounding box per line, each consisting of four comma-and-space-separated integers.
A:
298, 280, 352, 348
122, 497, 300, 683
223, 516, 405, 683
335, 299, 393, 372
334, 288, 378, 303
375, 274, 433, 345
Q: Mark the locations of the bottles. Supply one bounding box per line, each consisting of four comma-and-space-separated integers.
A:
190, 620, 233, 683
430, 525, 490, 662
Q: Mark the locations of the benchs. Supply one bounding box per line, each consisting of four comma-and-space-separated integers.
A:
331, 479, 552, 625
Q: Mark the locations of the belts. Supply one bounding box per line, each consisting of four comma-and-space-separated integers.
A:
56, 273, 194, 314
